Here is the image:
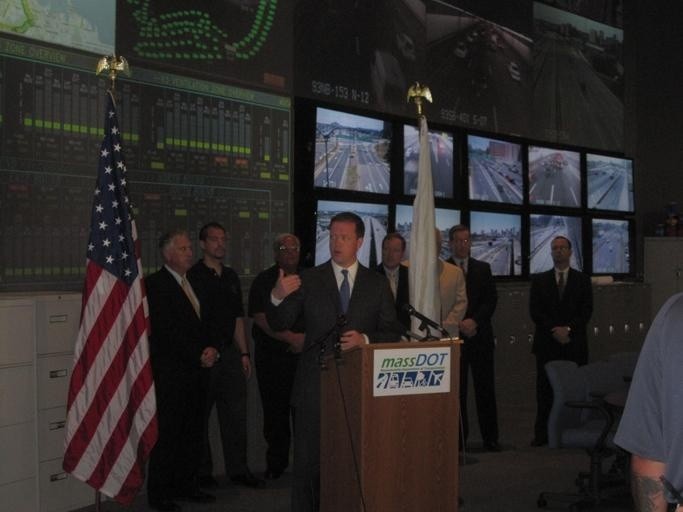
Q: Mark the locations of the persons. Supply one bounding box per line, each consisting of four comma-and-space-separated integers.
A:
184, 217, 267, 490
445, 223, 506, 453
265, 211, 401, 511
401, 224, 469, 339
526, 235, 593, 447
372, 230, 409, 337
611, 293, 682, 511
141, 227, 221, 512
245, 231, 306, 485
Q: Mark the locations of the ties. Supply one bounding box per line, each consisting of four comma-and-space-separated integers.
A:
181, 278, 199, 316
459, 260, 464, 274
558, 273, 565, 300
389, 273, 397, 298
339, 270, 349, 314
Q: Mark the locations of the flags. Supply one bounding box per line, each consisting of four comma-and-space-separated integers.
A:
408, 118, 444, 343
57, 88, 160, 507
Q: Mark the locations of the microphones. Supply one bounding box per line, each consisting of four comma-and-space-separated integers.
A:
401, 303, 450, 338
305, 313, 348, 355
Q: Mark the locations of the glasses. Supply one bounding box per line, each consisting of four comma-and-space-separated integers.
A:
278, 246, 298, 253
454, 238, 469, 244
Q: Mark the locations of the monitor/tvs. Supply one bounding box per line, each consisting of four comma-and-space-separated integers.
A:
399, 118, 467, 212
527, 139, 586, 218
291, 199, 394, 274
467, 130, 527, 214
2, 38, 308, 294
393, 203, 466, 274
117, 2, 297, 97
583, 215, 637, 283
525, 214, 586, 282
0, 0, 119, 64
425, 0, 533, 139
464, 208, 527, 282
535, 1, 629, 158
586, 148, 638, 216
298, 0, 427, 123
294, 99, 398, 198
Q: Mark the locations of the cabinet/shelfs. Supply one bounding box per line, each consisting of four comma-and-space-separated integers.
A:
493, 283, 537, 444
644, 237, 683, 329
593, 280, 650, 359
1, 294, 34, 511
34, 290, 109, 512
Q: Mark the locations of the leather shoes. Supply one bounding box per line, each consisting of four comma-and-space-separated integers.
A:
199, 475, 218, 491
264, 467, 283, 480
147, 494, 181, 512
229, 475, 265, 488
481, 442, 504, 452
529, 434, 547, 447
187, 490, 215, 503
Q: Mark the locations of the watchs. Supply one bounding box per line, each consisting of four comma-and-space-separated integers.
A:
566, 325, 572, 332
240, 351, 251, 359
216, 352, 220, 359
